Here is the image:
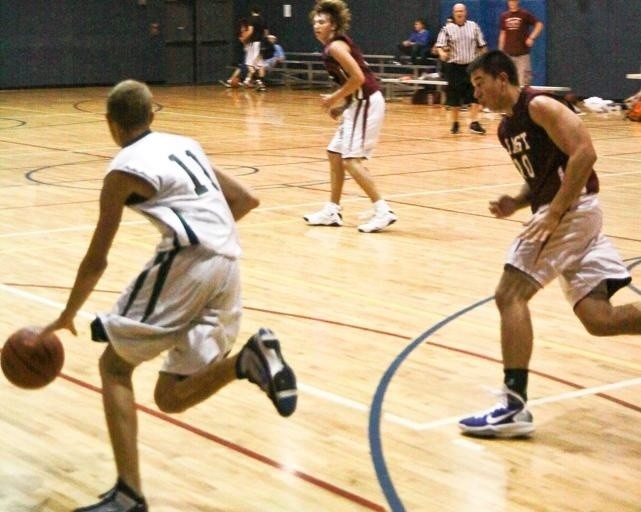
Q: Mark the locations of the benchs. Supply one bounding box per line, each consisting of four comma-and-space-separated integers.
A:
225, 49, 449, 103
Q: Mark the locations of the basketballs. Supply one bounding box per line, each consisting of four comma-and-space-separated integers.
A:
0, 325, 64, 389
232, 77, 241, 88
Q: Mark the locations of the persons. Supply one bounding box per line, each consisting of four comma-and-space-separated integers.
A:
498, 0, 544, 86
39, 81, 297, 512
435, 2, 488, 135
458, 48, 641, 440
302, 0, 397, 233
419, 16, 455, 67
218, 6, 285, 92
391, 20, 433, 66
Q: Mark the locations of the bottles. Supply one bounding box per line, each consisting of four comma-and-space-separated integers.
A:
428, 93, 436, 106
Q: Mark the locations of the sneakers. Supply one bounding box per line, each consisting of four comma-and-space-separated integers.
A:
69, 489, 148, 512
217, 77, 268, 91
359, 210, 398, 234
302, 208, 343, 227
450, 121, 459, 133
456, 407, 536, 435
238, 327, 298, 418
469, 121, 486, 134
